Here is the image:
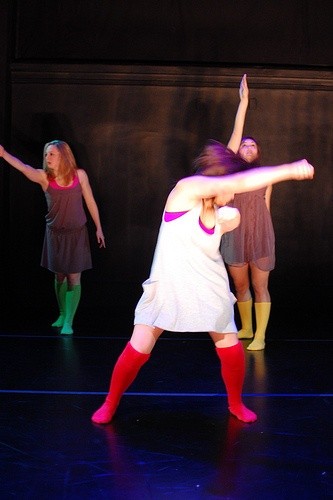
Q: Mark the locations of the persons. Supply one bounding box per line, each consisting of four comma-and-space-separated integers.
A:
0, 141, 107, 335
219, 74, 275, 351
91, 139, 314, 423
